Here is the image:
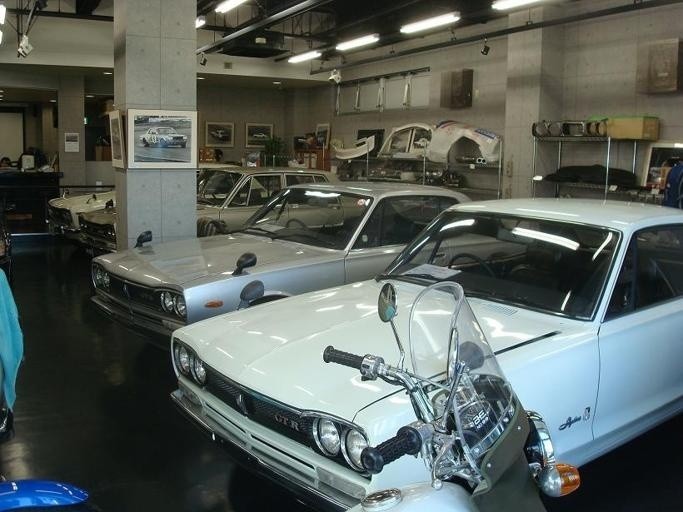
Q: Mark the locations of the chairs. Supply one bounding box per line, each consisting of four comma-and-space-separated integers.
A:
336, 216, 369, 244
507, 242, 564, 291
561, 251, 626, 306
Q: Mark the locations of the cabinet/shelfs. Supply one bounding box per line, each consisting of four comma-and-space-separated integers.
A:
295, 149, 331, 170
530, 136, 637, 200
351, 138, 503, 198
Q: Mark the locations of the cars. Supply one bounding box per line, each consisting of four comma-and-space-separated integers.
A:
254, 132, 267, 138
317, 136, 326, 145
165, 200, 682, 512
79, 152, 374, 261
84, 183, 481, 342
45, 164, 268, 244
211, 130, 229, 140
139, 125, 188, 150
0, 197, 19, 285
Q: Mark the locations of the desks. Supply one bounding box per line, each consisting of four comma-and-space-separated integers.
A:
0, 172, 64, 227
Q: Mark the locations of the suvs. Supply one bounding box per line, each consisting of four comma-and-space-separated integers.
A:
649, 156, 680, 191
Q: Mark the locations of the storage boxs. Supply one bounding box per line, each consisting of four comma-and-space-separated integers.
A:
606, 115, 658, 140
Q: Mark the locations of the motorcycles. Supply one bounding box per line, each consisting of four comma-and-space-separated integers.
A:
0, 472, 103, 512
302, 275, 586, 512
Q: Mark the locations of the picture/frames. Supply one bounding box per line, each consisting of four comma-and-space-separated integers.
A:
109, 110, 126, 168
127, 108, 198, 168
315, 122, 330, 149
245, 121, 274, 149
205, 120, 234, 148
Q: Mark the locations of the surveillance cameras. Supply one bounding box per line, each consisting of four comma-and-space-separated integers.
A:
481, 46, 489, 55
200, 51, 208, 66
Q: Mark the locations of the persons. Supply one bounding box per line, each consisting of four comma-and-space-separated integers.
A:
0, 157, 12, 167
661, 159, 683, 210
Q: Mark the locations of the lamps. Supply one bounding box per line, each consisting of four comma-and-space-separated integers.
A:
199, 52, 207, 64
481, 37, 490, 54
